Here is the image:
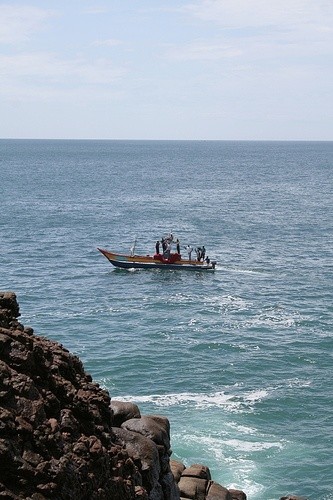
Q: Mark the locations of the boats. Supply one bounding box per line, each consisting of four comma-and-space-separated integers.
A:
98, 248, 217, 269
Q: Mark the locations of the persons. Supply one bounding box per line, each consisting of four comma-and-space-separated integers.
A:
184, 244, 210, 264
155, 233, 180, 256
